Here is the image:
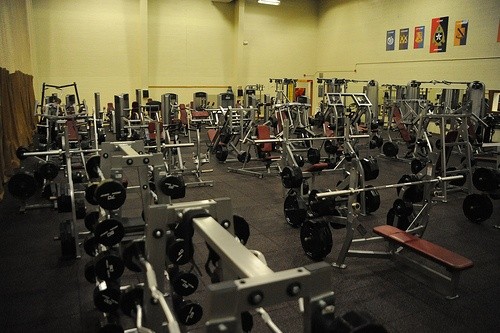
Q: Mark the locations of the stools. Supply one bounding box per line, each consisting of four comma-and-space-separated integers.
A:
371, 225, 475, 300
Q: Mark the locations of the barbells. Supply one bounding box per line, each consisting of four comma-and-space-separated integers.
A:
6, 109, 500, 333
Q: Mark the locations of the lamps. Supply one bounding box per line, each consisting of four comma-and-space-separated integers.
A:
257, 0, 280, 6
